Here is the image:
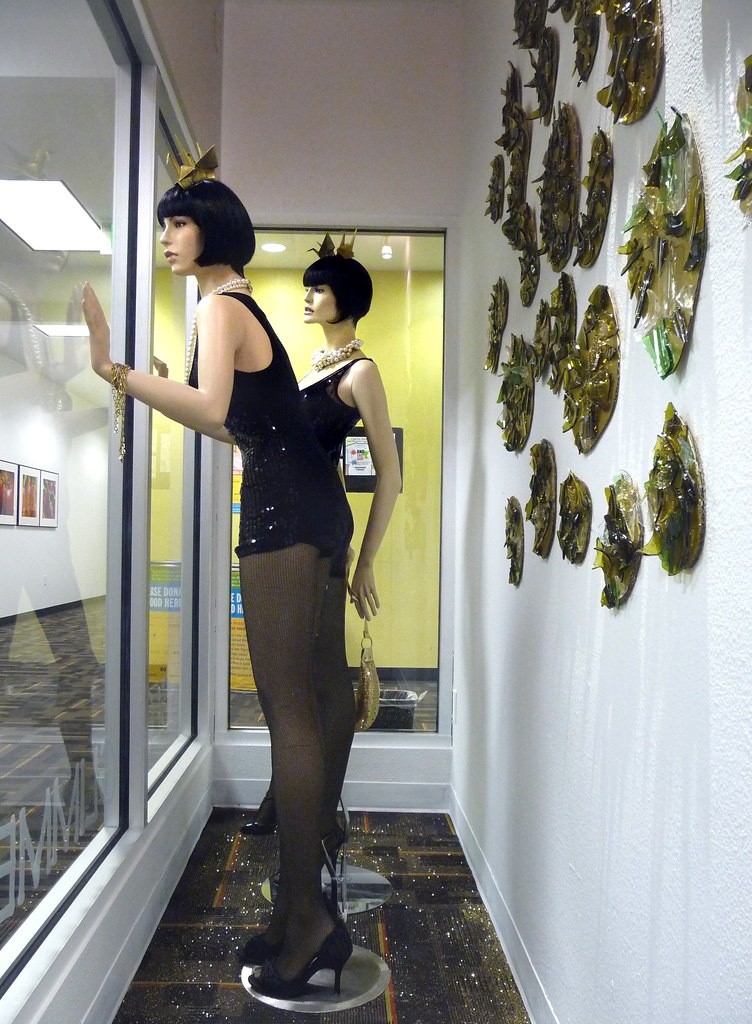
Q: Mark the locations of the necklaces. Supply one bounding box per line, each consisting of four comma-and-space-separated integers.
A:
184, 278, 254, 385
2, 280, 64, 413
310, 336, 365, 372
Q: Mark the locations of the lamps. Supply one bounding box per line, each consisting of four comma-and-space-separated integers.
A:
381, 236, 393, 260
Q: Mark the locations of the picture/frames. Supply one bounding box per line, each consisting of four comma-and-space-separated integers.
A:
1, 460, 59, 528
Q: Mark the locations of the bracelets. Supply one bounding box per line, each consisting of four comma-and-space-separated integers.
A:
111, 364, 130, 465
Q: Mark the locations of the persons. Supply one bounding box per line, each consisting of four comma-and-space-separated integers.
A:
239, 255, 401, 862
0, 197, 102, 816
79, 176, 357, 1001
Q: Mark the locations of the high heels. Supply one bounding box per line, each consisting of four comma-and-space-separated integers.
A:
270, 824, 344, 890
63, 775, 104, 811
234, 929, 293, 965
248, 919, 352, 999
240, 795, 279, 835
